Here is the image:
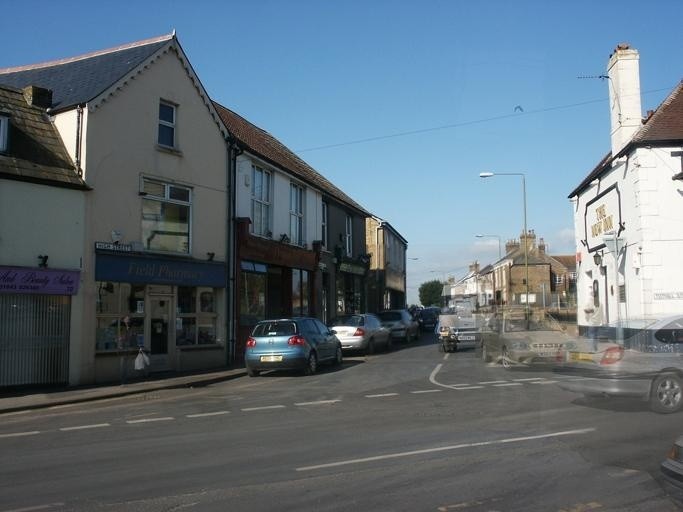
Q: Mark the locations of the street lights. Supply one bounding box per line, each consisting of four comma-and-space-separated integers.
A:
475, 234, 500, 260
479, 172, 529, 329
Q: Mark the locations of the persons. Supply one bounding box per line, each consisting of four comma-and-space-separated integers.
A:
119, 314, 145, 386
435, 305, 456, 334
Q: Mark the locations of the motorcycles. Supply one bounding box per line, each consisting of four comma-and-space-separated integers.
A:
439, 323, 459, 352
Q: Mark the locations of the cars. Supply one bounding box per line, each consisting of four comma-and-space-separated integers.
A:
327, 313, 392, 354
244, 316, 342, 378
377, 309, 419, 343
419, 307, 441, 330
480, 305, 576, 370
660, 434, 683, 501
434, 307, 483, 348
552, 313, 683, 412
